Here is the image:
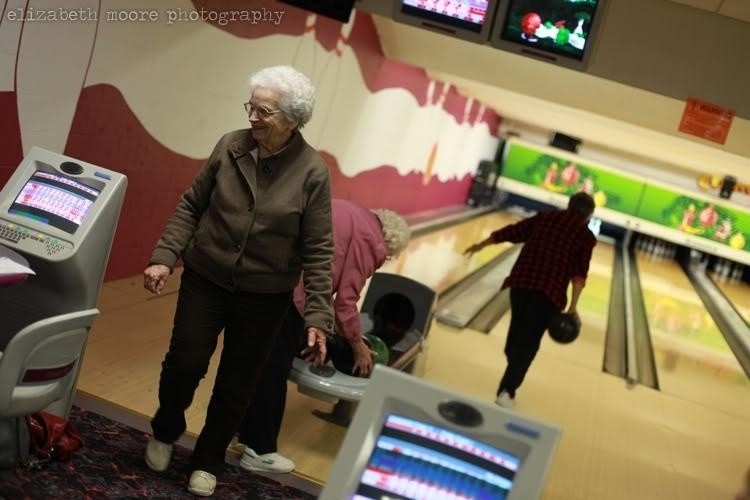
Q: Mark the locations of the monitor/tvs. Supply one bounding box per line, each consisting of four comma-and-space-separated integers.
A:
9, 169, 99, 235
391, 0, 497, 46
492, 0, 616, 70
343, 413, 520, 500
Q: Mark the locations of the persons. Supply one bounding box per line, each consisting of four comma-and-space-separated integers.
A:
461, 189, 597, 409
291, 199, 411, 380
138, 62, 335, 498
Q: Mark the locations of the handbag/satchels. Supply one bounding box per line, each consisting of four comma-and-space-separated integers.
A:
24, 411, 85, 463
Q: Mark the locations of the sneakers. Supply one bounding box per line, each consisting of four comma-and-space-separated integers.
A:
145, 438, 173, 474
224, 443, 245, 466
240, 447, 295, 474
494, 389, 514, 411
189, 471, 216, 497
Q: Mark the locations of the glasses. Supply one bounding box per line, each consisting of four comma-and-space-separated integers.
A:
245, 103, 284, 116
